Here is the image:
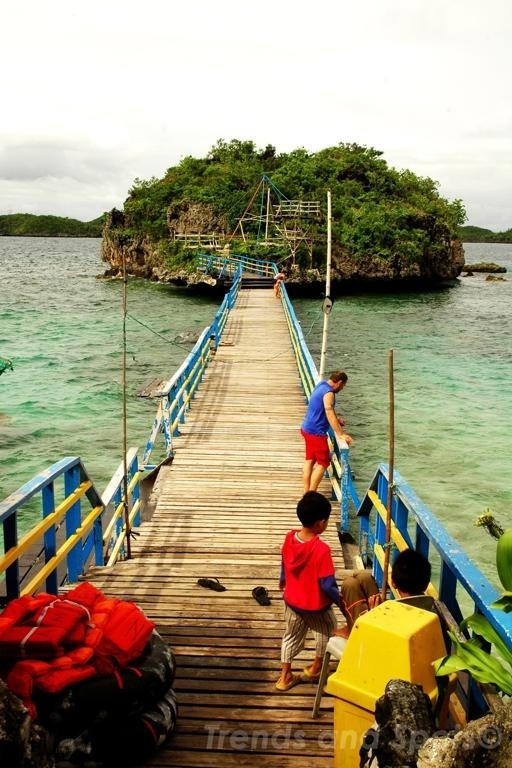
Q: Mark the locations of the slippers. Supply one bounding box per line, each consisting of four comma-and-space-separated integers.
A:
253, 587, 270, 606
197, 577, 225, 592
275, 672, 301, 691
304, 664, 330, 677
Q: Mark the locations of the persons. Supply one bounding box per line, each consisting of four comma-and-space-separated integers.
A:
342, 550, 452, 659
276, 491, 343, 691
301, 371, 353, 491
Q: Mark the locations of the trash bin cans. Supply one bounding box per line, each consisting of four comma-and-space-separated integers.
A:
326, 599, 458, 768
